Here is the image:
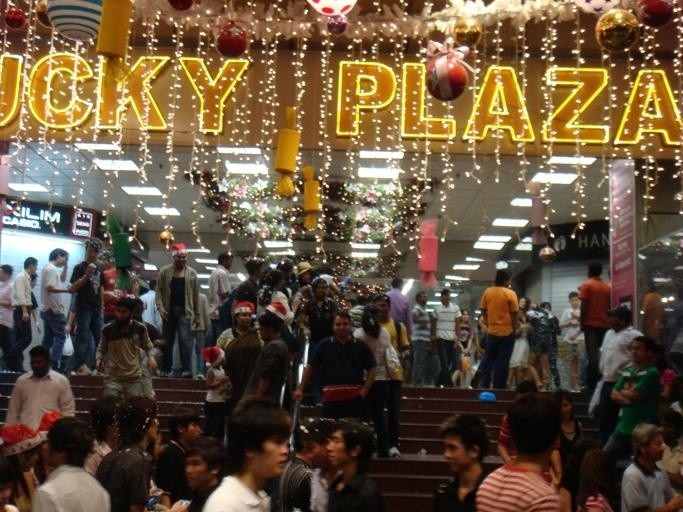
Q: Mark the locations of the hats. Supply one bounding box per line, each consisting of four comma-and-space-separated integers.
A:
296, 262, 314, 276
37, 411, 62, 440
200, 346, 225, 368
604, 306, 632, 321
171, 243, 187, 257
265, 302, 295, 322
233, 301, 256, 320
84, 236, 104, 254
0, 423, 42, 458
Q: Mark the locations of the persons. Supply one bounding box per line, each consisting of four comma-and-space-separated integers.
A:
1, 232, 683, 511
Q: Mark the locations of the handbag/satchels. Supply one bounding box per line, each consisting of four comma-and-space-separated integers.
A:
384, 344, 401, 374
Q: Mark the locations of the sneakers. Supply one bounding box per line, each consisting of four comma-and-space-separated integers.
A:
387, 446, 400, 457
159, 370, 168, 378
181, 371, 191, 378
196, 373, 204, 379
